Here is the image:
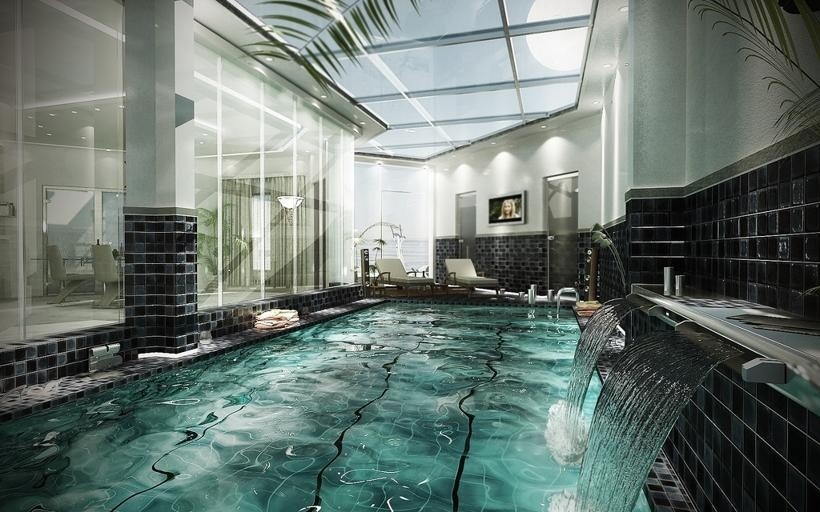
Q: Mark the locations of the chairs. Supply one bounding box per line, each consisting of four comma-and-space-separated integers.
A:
46, 243, 124, 309
374, 258, 500, 298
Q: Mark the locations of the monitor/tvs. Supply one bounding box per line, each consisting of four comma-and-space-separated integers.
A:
488, 190, 525, 226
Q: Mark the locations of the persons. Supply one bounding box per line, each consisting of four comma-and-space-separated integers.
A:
497, 199, 519, 220
